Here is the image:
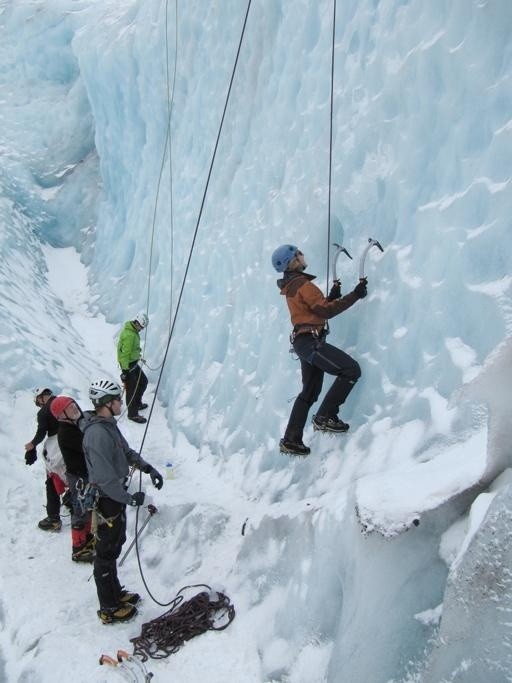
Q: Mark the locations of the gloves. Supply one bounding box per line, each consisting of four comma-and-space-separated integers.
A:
354, 280, 368, 298
123, 370, 133, 377
150, 468, 163, 490
130, 492, 145, 506
328, 282, 342, 301
25, 447, 37, 465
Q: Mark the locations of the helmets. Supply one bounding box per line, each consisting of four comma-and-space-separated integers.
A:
51, 396, 74, 419
32, 385, 53, 406
272, 244, 299, 273
89, 378, 122, 400
134, 312, 149, 328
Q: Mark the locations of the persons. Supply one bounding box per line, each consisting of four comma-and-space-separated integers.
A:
117, 312, 149, 422
50, 396, 97, 562
271, 244, 368, 454
24, 387, 66, 531
81, 378, 163, 623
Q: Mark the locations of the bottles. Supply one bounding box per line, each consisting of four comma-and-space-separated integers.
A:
166, 463, 174, 480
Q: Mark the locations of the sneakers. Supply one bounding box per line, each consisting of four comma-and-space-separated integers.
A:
137, 404, 147, 410
127, 414, 147, 423
38, 514, 62, 532
279, 438, 310, 456
101, 601, 137, 624
313, 412, 349, 432
118, 590, 141, 605
72, 534, 96, 562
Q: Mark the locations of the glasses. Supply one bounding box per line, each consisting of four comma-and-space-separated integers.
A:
294, 251, 303, 257
111, 396, 122, 401
